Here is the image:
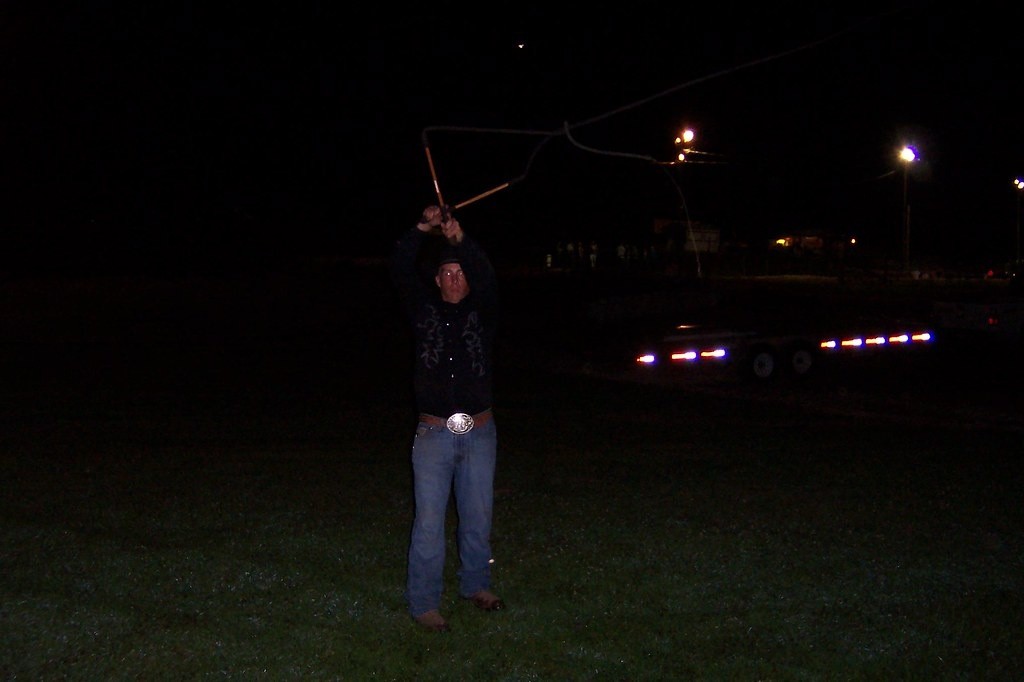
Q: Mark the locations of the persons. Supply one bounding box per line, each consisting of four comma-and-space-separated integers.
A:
401, 204, 507, 630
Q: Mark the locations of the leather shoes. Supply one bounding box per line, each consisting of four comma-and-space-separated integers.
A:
411, 609, 451, 633
458, 589, 506, 612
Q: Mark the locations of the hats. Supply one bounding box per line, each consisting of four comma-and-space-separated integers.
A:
430, 245, 463, 269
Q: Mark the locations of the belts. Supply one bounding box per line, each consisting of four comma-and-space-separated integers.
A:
419, 409, 492, 435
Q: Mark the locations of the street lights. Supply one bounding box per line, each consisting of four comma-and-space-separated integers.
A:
901, 147, 916, 268
